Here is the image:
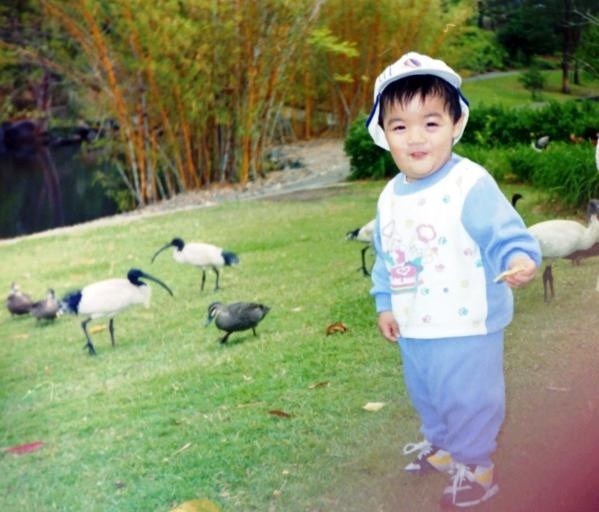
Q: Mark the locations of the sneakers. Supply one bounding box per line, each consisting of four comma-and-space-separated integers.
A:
403, 440, 451, 478
439, 463, 497, 509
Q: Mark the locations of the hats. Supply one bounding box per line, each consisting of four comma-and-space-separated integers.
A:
365, 50, 470, 150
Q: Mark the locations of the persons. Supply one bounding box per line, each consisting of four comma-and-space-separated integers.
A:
362, 50, 548, 512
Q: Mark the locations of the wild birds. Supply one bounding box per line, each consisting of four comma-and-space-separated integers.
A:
205, 302, 272, 344
7, 281, 61, 325
151, 238, 239, 293
344, 218, 376, 277
59, 268, 174, 356
527, 198, 599, 302
